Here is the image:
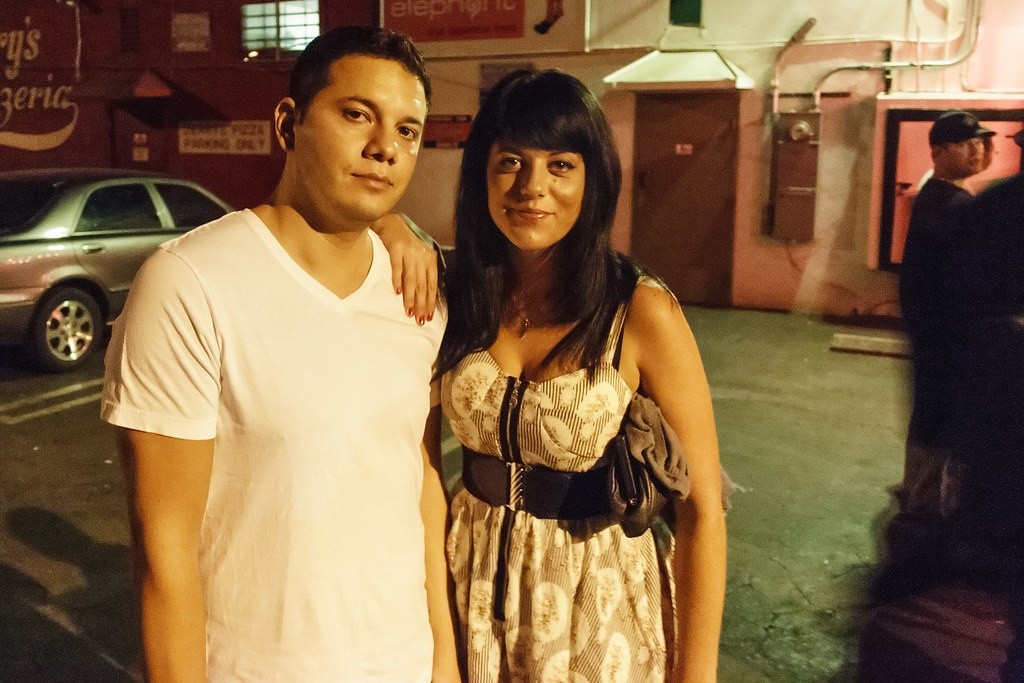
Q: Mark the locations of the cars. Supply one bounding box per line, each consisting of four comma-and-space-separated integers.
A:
0, 167, 241, 374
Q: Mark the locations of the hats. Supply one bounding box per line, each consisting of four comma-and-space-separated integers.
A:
927, 112, 994, 147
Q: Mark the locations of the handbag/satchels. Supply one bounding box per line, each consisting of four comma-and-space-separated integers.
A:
610, 394, 686, 539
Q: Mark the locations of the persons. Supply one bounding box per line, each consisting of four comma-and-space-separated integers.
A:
843, 158, 1024, 682
889, 112, 999, 505
97, 23, 455, 680
361, 64, 742, 682
913, 131, 993, 204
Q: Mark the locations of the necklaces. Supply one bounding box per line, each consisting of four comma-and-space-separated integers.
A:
504, 288, 551, 330
